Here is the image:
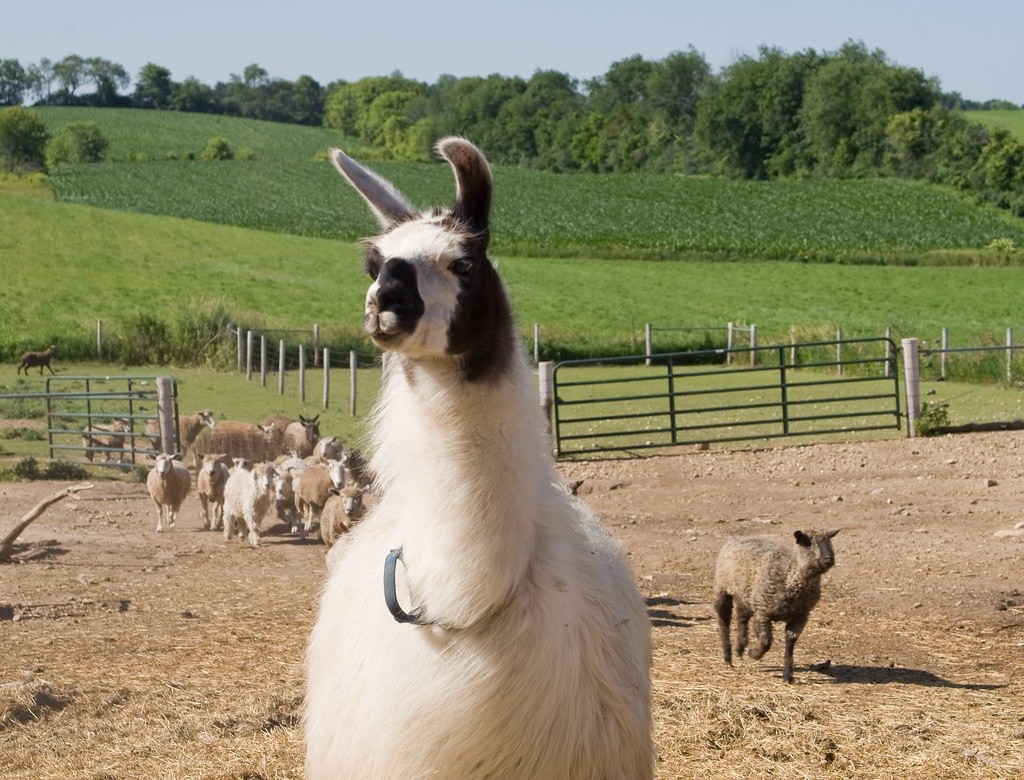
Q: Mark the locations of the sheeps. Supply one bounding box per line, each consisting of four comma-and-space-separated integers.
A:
714, 527, 843, 686
16, 344, 58, 376
79, 410, 382, 549
